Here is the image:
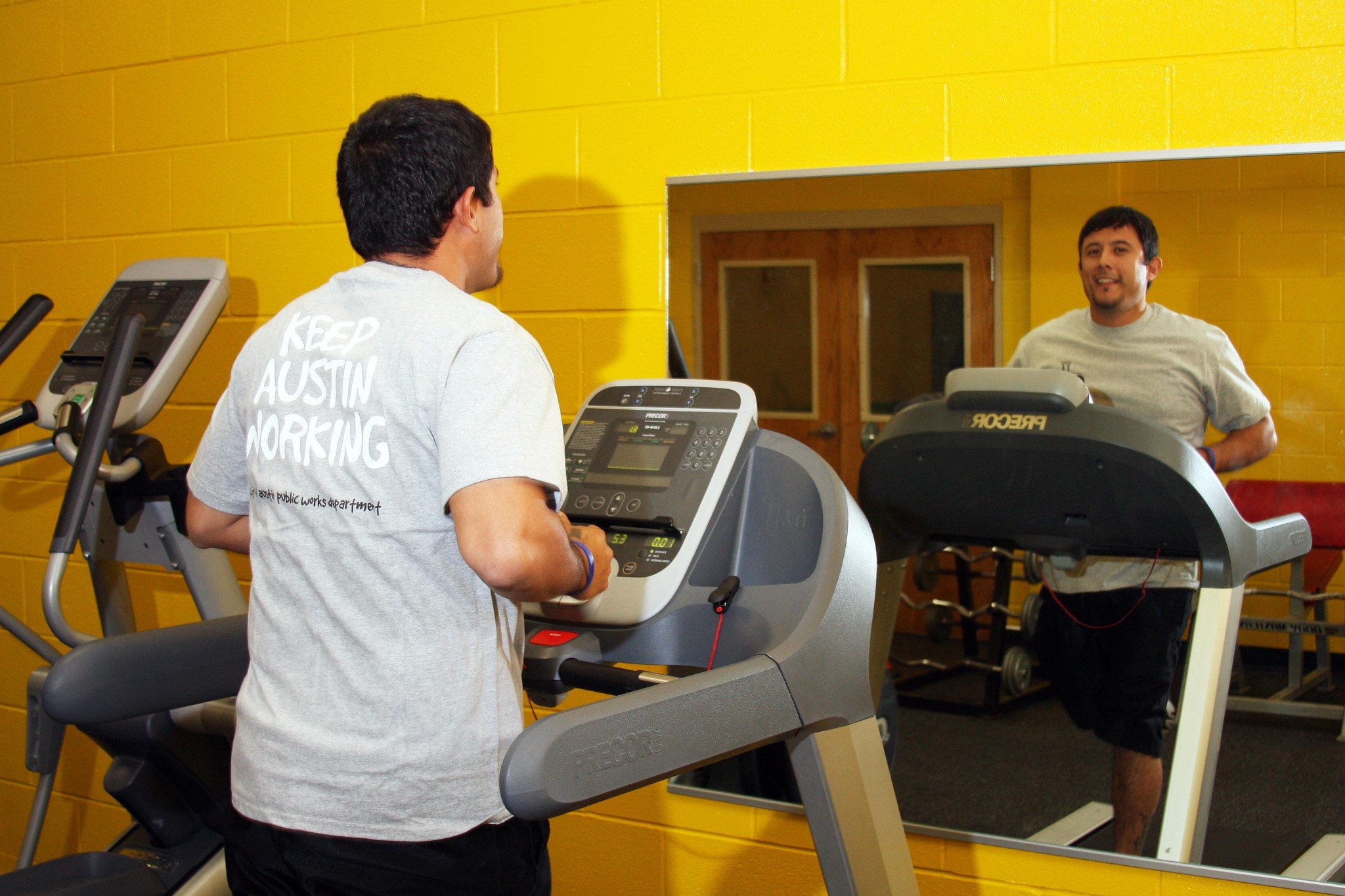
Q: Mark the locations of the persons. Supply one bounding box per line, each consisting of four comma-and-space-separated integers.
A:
173, 89, 618, 895
1006, 205, 1280, 861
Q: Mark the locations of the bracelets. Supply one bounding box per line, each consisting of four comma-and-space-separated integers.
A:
1199, 446, 1216, 471
568, 538, 595, 597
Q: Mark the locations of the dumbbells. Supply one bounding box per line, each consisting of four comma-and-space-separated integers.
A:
886, 633, 1032, 701
913, 541, 1047, 589
921, 589, 1043, 643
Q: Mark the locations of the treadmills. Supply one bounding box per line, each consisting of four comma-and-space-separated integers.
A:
0, 375, 925, 895
855, 364, 1312, 868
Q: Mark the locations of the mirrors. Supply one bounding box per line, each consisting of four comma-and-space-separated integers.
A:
659, 139, 1345, 893
